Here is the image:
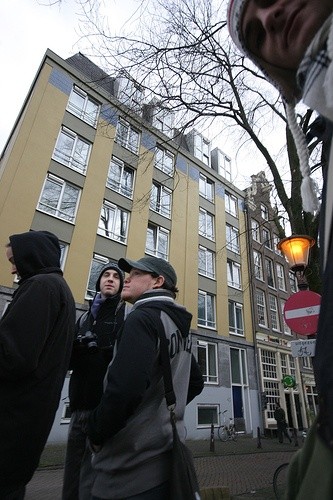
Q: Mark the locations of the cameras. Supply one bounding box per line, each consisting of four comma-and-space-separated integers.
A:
80, 334, 98, 348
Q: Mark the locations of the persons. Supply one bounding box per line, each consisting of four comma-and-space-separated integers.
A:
91, 257, 206, 500
61, 263, 135, 500
0, 230, 76, 500
274, 403, 291, 443
229, 0, 332, 500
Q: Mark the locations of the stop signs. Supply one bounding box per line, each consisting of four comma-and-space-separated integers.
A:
283, 291, 325, 336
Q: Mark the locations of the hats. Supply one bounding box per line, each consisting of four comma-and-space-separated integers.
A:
118, 256, 177, 288
91, 263, 125, 304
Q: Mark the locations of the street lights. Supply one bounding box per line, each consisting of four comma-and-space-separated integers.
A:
277, 232, 316, 380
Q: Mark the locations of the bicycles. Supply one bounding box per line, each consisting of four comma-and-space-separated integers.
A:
218, 410, 238, 442
273, 429, 310, 500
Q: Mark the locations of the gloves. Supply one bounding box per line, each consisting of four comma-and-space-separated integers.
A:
100, 346, 113, 362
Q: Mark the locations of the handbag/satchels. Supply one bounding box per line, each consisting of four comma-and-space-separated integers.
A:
88, 439, 200, 500
